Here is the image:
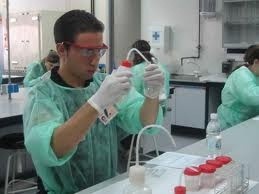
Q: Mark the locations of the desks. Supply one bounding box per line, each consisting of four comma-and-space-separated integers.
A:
0, 87, 37, 186
75, 115, 259, 194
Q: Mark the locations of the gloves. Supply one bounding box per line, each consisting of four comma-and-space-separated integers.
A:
142, 63, 165, 100
87, 69, 133, 116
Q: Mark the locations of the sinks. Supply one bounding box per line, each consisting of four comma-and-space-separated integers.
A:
169, 76, 202, 81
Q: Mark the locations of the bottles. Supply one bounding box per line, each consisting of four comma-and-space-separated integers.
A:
206, 114, 223, 159
143, 62, 160, 99
116, 165, 153, 194
117, 60, 133, 73
174, 155, 232, 194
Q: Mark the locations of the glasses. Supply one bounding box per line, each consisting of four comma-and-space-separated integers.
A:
58, 40, 109, 57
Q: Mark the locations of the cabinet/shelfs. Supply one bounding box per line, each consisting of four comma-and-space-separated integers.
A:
167, 84, 221, 130
221, 0, 259, 50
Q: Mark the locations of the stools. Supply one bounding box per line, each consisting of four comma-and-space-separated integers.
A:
0, 133, 38, 194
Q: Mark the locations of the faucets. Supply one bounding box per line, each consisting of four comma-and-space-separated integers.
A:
187, 59, 200, 75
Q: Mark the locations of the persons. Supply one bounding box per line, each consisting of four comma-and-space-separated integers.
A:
217, 45, 259, 129
23, 49, 60, 89
116, 40, 171, 158
23, 10, 165, 194
